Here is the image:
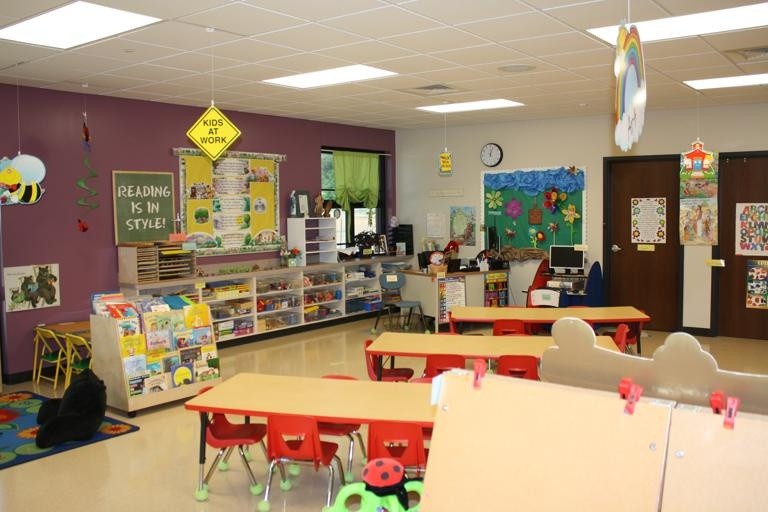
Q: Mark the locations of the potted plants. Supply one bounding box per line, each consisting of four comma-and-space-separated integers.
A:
351, 230, 380, 258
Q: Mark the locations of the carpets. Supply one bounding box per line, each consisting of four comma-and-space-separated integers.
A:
0, 390, 140, 471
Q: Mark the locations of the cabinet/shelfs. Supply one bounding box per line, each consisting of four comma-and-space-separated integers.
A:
117, 246, 408, 343
286, 217, 337, 267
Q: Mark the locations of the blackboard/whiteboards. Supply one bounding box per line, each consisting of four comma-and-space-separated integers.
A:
112, 170, 176, 247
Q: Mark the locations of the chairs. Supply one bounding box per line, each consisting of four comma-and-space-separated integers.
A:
36, 327, 94, 392
370, 272, 431, 334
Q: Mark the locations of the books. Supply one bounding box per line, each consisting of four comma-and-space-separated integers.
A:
90, 291, 221, 395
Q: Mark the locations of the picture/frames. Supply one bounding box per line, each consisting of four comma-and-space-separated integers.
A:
295, 191, 310, 216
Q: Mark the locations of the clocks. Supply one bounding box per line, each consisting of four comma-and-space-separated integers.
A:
479, 143, 503, 168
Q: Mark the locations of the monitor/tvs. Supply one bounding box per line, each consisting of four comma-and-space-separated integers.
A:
549, 245, 584, 275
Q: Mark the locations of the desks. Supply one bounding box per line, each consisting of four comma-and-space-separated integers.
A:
32, 320, 90, 383
